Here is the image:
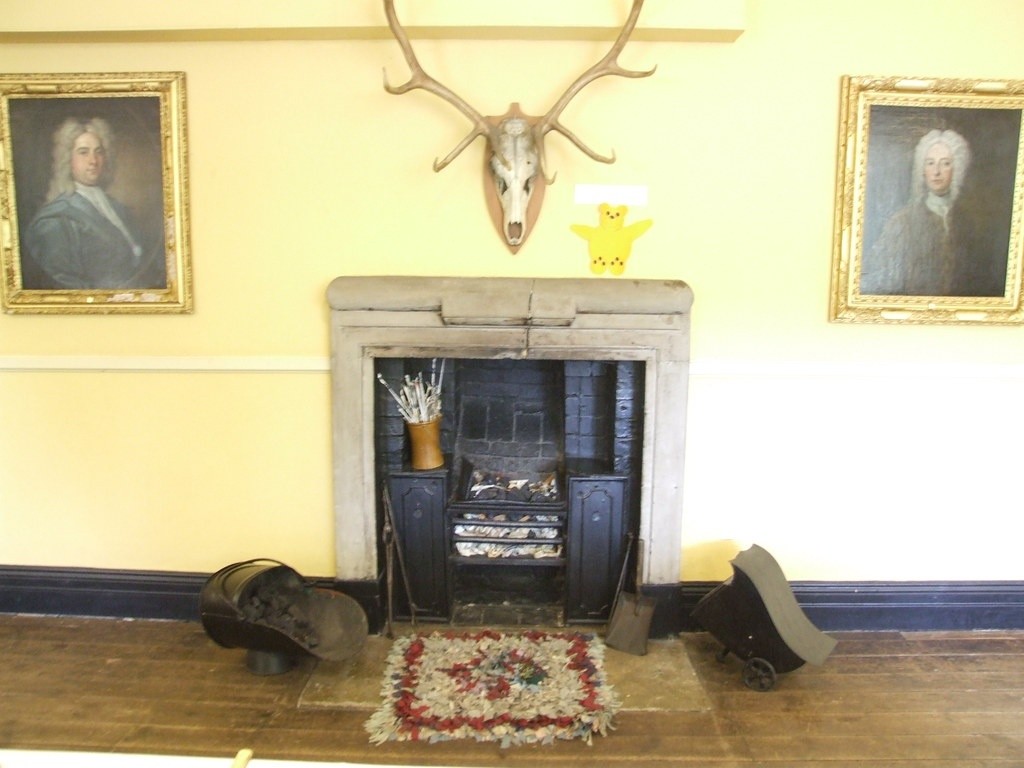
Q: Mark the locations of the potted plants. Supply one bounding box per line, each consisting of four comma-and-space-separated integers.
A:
375, 356, 450, 470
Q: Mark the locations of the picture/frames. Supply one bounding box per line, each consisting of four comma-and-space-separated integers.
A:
830, 75, 1024, 324
1, 70, 195, 317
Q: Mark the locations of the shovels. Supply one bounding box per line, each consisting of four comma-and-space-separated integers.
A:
603, 541, 658, 656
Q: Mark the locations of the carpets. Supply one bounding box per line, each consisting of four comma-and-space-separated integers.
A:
364, 628, 618, 749
297, 632, 711, 717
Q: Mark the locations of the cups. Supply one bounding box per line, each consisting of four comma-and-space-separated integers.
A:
407, 415, 445, 469
